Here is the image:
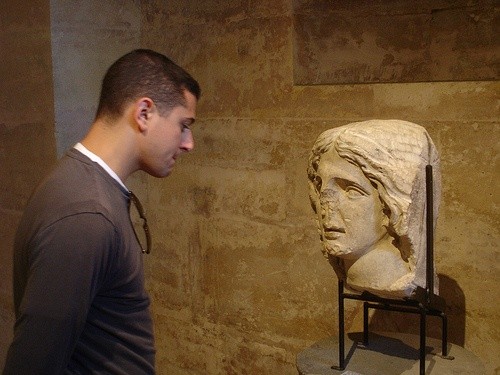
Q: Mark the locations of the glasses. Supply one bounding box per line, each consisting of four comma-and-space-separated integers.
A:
123, 187, 153, 257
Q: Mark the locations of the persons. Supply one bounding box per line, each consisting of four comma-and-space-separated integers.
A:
304, 116, 442, 302
0, 49, 203, 375
